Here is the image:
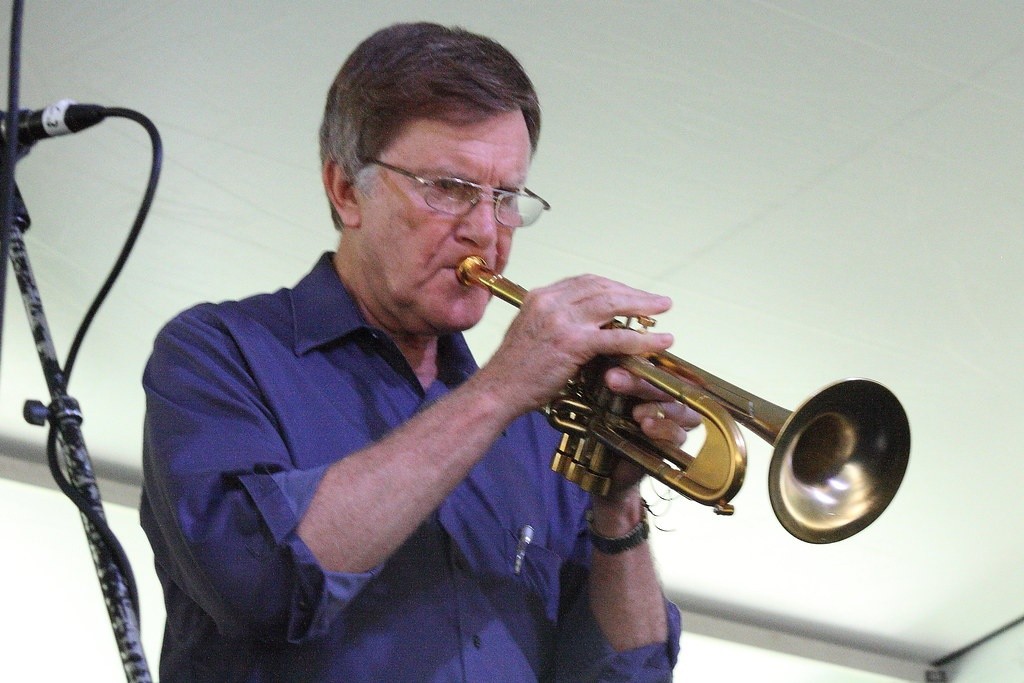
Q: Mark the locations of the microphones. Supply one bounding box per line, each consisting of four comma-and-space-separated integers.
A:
0, 101, 108, 165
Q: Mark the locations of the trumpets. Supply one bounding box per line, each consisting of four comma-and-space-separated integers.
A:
456, 253, 913, 546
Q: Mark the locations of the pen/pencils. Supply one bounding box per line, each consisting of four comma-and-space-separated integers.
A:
514, 525, 534, 575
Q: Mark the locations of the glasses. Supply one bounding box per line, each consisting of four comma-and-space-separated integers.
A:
367, 157, 551, 230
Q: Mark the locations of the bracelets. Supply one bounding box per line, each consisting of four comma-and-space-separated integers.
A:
586, 493, 649, 554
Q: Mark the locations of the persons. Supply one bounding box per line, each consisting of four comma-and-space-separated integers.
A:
139, 23, 702, 683
